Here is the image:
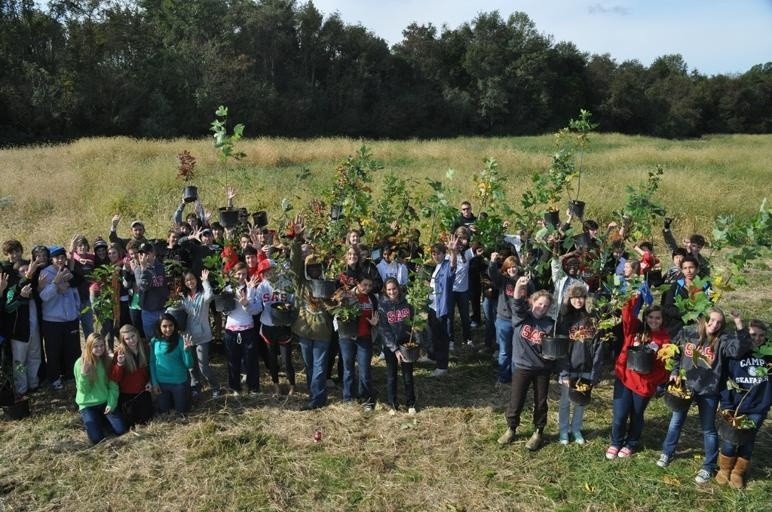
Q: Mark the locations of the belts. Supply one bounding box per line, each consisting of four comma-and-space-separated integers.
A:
347, 335, 367, 340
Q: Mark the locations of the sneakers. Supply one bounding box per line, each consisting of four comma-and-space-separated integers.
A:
408, 407, 416, 416
426, 367, 449, 378
618, 446, 636, 458
300, 400, 324, 411
326, 378, 336, 387
389, 408, 397, 416
525, 431, 543, 451
448, 315, 502, 356
606, 445, 621, 460
559, 433, 569, 445
496, 427, 515, 444
656, 454, 674, 467
694, 470, 712, 483
572, 430, 585, 445
18, 376, 64, 396
361, 400, 375, 418
191, 384, 297, 399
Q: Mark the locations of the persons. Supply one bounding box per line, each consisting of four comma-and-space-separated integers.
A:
1, 192, 204, 445
712, 316, 772, 491
424, 198, 713, 448
602, 290, 673, 460
201, 186, 417, 415
653, 302, 746, 486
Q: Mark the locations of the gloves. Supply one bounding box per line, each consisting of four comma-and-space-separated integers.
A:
664, 217, 672, 230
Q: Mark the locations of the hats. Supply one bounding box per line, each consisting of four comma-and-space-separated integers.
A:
567, 283, 587, 298
93, 236, 108, 251
131, 220, 144, 228
239, 207, 251, 217
137, 242, 153, 253
48, 245, 66, 257
202, 227, 212, 236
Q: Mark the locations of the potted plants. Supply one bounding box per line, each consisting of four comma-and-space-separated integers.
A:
198, 255, 237, 313
238, 166, 272, 228
257, 251, 299, 328
159, 256, 189, 331
177, 150, 200, 202
208, 105, 248, 229
0, 360, 32, 420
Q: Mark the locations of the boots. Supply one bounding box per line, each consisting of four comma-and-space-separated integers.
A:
731, 457, 750, 489
715, 452, 736, 486
